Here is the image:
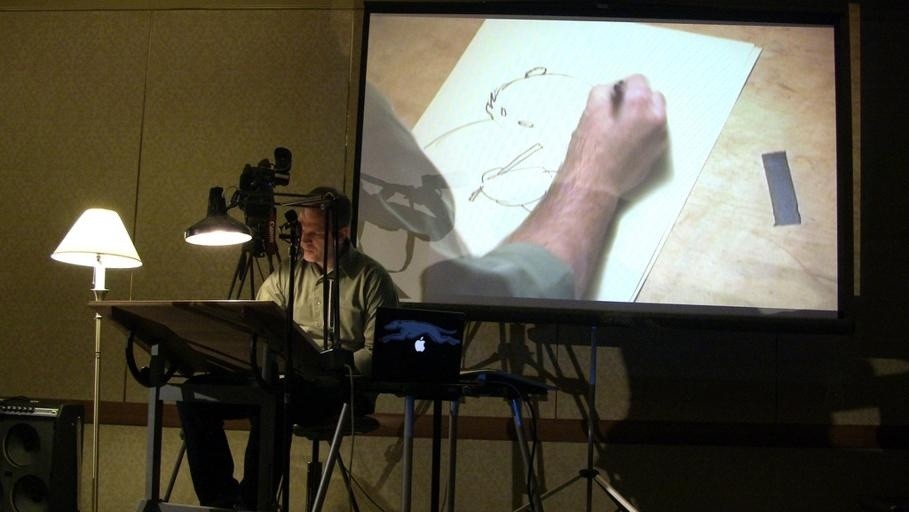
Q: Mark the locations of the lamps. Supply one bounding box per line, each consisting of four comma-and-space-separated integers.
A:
183, 184, 353, 382
49, 204, 144, 512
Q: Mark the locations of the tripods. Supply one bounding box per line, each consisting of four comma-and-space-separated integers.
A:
511, 325, 640, 512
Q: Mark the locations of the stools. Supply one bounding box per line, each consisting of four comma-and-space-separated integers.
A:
293, 410, 381, 512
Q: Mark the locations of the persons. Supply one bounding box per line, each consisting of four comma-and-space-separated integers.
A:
392, 74, 668, 301
177, 187, 400, 512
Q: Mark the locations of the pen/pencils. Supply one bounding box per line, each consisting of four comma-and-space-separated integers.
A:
613, 84, 624, 104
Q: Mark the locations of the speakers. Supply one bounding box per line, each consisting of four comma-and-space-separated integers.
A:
0, 416, 83, 512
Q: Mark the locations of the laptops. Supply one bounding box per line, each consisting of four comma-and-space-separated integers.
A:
372, 306, 466, 383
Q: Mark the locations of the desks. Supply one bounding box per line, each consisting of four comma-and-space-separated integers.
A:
85, 300, 347, 512
